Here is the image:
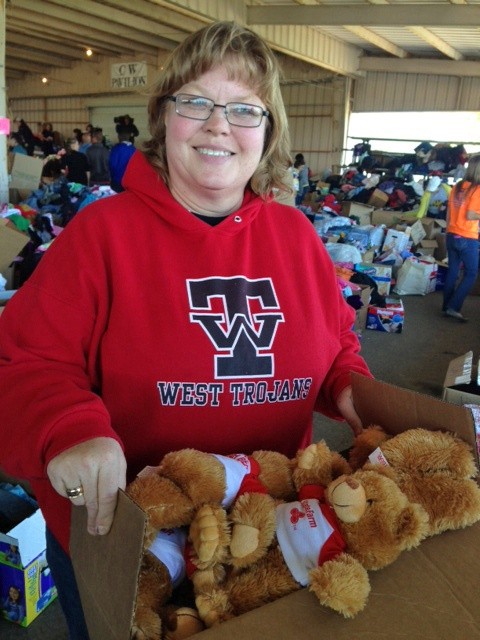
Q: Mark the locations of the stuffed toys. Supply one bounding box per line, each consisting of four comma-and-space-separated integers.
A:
186, 473, 428, 626
121, 448, 296, 548
156, 588, 202, 638
112, 509, 192, 640
295, 424, 480, 538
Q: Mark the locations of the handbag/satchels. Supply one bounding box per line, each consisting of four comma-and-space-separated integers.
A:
392, 256, 438, 296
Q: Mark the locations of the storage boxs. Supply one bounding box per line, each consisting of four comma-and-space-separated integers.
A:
11, 153, 45, 201
66, 370, 479, 639
357, 263, 393, 294
347, 284, 372, 330
300, 176, 448, 258
365, 297, 403, 334
443, 350, 480, 448
0, 217, 31, 273
0, 473, 59, 627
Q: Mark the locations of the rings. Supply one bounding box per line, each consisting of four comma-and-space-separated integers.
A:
66, 487, 83, 498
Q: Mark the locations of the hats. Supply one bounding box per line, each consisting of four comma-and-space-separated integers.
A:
427, 176, 442, 193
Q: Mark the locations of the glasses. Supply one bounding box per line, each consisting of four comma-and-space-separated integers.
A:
165, 93, 270, 129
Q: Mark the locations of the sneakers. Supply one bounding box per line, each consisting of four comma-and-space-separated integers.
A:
444, 312, 448, 315
449, 310, 469, 322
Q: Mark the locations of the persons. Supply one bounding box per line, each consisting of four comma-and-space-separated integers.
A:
0, 22, 382, 640
120, 115, 139, 146
86, 128, 112, 185
42, 123, 54, 150
13, 116, 36, 155
288, 160, 305, 206
441, 154, 479, 321
63, 136, 91, 190
294, 153, 309, 205
72, 129, 83, 150
108, 126, 137, 194
43, 148, 68, 181
6, 132, 28, 159
85, 124, 94, 140
